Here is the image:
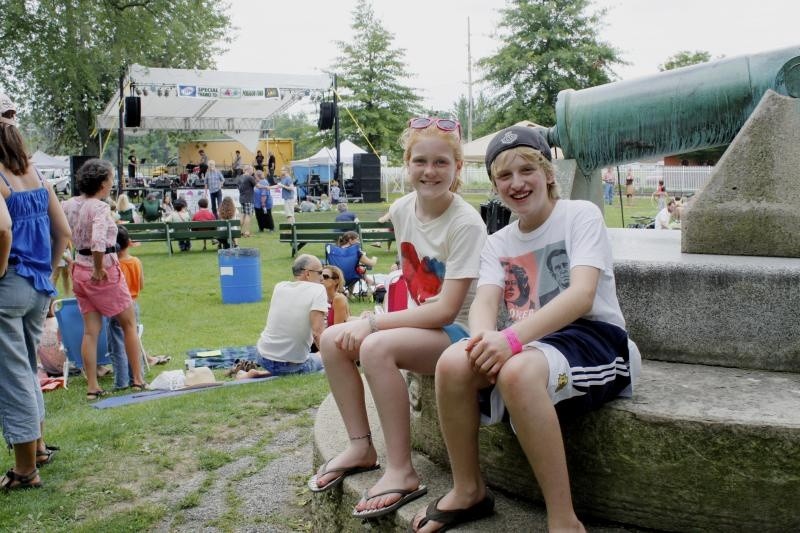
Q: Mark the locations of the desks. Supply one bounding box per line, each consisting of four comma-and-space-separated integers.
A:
111, 188, 164, 202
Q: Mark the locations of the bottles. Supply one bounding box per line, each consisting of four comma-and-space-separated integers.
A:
263, 207, 268, 214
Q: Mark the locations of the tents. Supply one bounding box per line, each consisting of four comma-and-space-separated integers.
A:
96, 63, 341, 207
288, 140, 337, 205
30, 150, 66, 184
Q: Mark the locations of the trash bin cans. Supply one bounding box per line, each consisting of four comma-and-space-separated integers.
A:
218, 247, 263, 303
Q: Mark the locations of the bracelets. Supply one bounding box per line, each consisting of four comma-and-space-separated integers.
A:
368, 314, 378, 333
502, 328, 523, 355
282, 185, 284, 188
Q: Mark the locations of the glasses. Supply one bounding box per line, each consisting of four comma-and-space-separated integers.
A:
299, 268, 323, 276
408, 118, 462, 143
322, 274, 339, 281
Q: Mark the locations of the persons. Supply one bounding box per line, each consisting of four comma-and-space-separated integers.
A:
603, 164, 687, 230
0, 93, 73, 492
334, 202, 362, 243
62, 158, 152, 400
339, 231, 378, 292
1, 191, 12, 278
379, 209, 397, 251
236, 254, 332, 378
407, 126, 642, 533
311, 266, 349, 357
308, 118, 488, 519
100, 150, 341, 251
52, 197, 74, 300
112, 224, 145, 391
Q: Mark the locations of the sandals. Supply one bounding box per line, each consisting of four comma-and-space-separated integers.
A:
87, 388, 110, 401
36, 447, 54, 465
0, 467, 41, 491
131, 382, 154, 393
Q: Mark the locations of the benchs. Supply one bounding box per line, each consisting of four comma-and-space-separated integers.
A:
121, 220, 242, 258
279, 222, 395, 257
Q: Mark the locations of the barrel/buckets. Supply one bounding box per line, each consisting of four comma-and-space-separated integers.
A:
218, 247, 262, 305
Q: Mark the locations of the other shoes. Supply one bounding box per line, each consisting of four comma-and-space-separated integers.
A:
232, 358, 257, 372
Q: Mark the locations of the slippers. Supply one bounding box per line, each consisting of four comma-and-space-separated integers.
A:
407, 488, 494, 533
353, 484, 427, 518
97, 370, 114, 378
152, 356, 167, 365
308, 455, 381, 491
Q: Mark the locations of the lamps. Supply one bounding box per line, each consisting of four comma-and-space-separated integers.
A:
136, 87, 169, 96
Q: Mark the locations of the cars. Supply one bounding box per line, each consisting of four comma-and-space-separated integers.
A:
37, 169, 70, 196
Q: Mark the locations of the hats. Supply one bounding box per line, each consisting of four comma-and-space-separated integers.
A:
486, 126, 552, 179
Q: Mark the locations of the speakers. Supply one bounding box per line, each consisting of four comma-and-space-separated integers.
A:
126, 97, 140, 127
318, 102, 334, 130
70, 156, 98, 198
353, 154, 380, 202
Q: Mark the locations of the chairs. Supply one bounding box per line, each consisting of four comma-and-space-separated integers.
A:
52, 299, 150, 389
325, 244, 376, 303
